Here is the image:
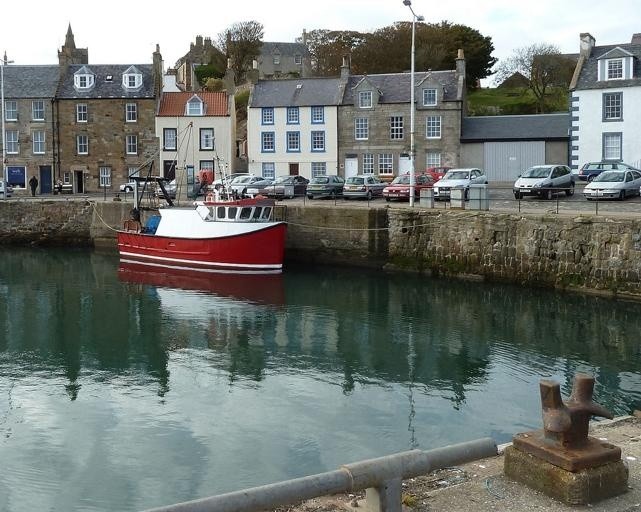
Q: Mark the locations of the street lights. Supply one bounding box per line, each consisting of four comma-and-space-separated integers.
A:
402, 0, 425, 207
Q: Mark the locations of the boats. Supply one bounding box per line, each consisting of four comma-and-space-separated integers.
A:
112, 121, 288, 275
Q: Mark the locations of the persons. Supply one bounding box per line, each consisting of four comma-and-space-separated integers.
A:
55, 178, 63, 197
112, 193, 122, 202
28, 174, 40, 197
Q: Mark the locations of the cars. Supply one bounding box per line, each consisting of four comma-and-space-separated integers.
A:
0, 180, 14, 198
157, 179, 176, 199
211, 168, 488, 201
513, 165, 576, 200
119, 176, 168, 199
578, 160, 641, 201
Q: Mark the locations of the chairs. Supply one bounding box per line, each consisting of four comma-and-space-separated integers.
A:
141, 214, 161, 234
